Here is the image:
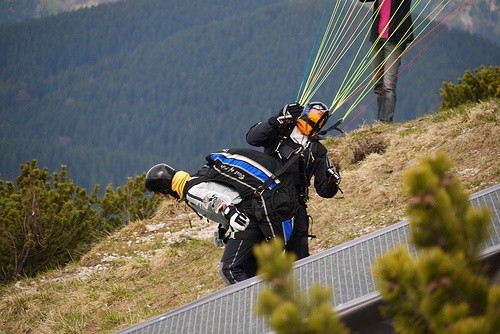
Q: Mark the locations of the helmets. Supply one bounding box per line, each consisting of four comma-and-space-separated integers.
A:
145, 163, 176, 194
305, 101, 330, 116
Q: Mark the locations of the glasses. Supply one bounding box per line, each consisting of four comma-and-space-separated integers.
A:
312, 105, 324, 110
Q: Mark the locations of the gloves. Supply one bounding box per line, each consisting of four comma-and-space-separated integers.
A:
268, 102, 304, 130
326, 166, 341, 187
214, 224, 229, 247
224, 206, 253, 233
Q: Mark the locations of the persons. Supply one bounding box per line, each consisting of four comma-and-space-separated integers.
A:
358, 0, 414, 125
144, 163, 266, 286
246, 101, 342, 262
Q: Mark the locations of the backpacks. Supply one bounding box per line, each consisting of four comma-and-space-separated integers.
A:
184, 149, 298, 248
